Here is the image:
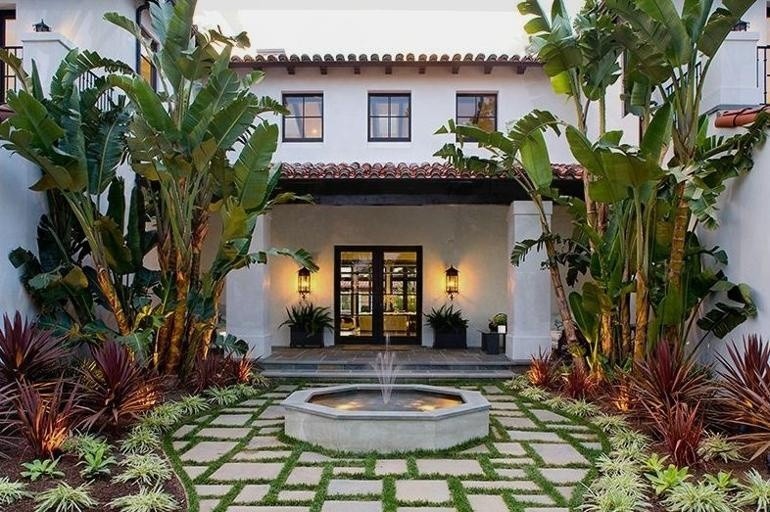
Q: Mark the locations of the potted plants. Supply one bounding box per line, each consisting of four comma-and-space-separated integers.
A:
423, 304, 468, 348
489, 313, 507, 332
278, 303, 334, 347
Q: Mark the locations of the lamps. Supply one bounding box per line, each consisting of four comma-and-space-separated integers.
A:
298, 266, 311, 298
445, 264, 459, 300
